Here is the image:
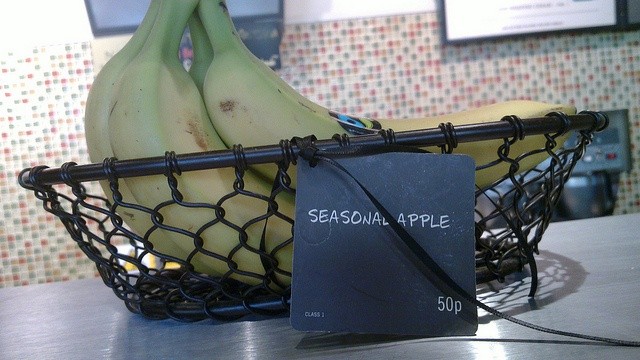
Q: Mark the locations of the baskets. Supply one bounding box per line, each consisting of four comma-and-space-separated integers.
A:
18, 109, 610, 322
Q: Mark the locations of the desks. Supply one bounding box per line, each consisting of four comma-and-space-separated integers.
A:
1, 211, 640, 359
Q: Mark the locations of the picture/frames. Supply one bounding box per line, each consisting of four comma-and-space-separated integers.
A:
83, 0, 283, 37
435, 0, 628, 47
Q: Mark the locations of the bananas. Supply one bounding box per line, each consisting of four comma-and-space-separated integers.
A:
84, 0, 578, 291
